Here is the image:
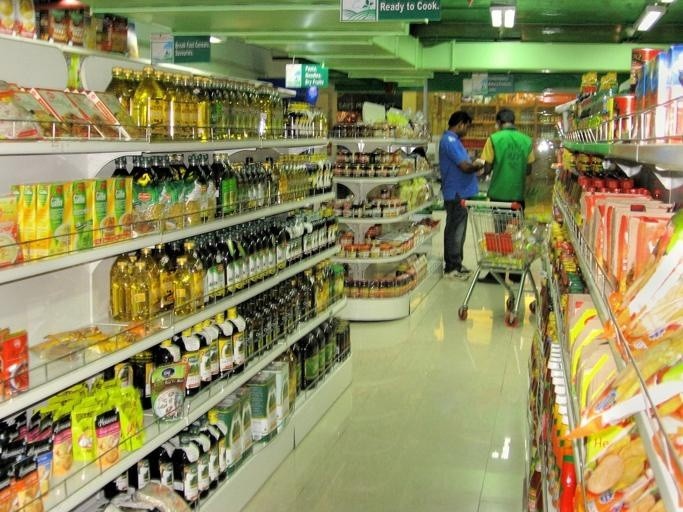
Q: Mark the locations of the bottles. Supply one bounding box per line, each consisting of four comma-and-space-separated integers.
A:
104, 66, 351, 509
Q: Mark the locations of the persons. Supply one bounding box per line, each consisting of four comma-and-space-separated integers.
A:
435, 110, 484, 280
476, 108, 535, 287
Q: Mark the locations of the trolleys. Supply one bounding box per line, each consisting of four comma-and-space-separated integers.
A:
458, 198, 547, 325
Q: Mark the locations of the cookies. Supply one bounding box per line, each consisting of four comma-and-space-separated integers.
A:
585, 437, 647, 494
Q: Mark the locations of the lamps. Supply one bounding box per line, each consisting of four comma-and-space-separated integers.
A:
632, 4, 667, 32
488, 4, 515, 29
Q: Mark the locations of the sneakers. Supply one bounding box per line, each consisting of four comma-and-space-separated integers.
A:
444, 264, 474, 282
478, 277, 504, 284
501, 273, 520, 283
